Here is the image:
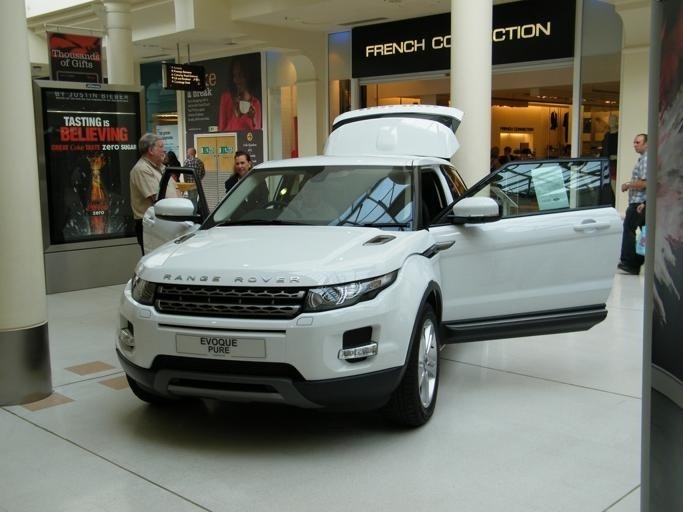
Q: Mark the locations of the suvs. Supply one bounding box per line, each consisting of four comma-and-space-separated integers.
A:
116, 104, 624, 429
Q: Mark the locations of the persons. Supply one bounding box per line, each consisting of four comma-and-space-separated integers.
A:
225, 151, 269, 220
129, 133, 179, 256
182, 147, 205, 202
618, 134, 648, 275
167, 151, 181, 182
491, 146, 513, 174
218, 61, 261, 132
